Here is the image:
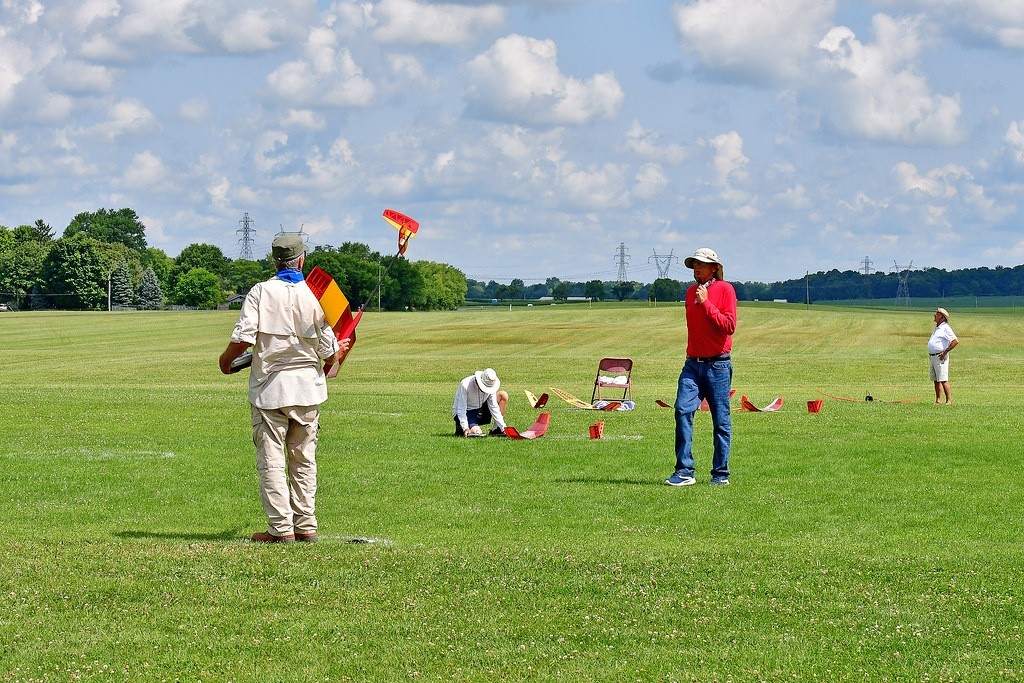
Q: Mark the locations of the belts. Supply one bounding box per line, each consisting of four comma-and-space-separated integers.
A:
929, 353, 939, 357
689, 356, 731, 362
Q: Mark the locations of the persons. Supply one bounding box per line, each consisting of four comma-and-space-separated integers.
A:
220, 236, 351, 542
928, 307, 959, 404
452, 368, 509, 438
664, 248, 736, 487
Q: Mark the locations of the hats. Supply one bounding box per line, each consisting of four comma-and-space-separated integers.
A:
271, 234, 309, 260
684, 248, 723, 282
475, 368, 500, 394
933, 307, 949, 322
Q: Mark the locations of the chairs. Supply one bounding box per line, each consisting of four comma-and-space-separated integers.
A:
591, 357, 633, 405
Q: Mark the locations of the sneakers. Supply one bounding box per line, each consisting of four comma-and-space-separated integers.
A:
708, 475, 730, 486
295, 531, 317, 542
664, 471, 696, 487
251, 531, 295, 542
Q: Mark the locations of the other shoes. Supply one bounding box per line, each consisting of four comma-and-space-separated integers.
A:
488, 426, 506, 437
